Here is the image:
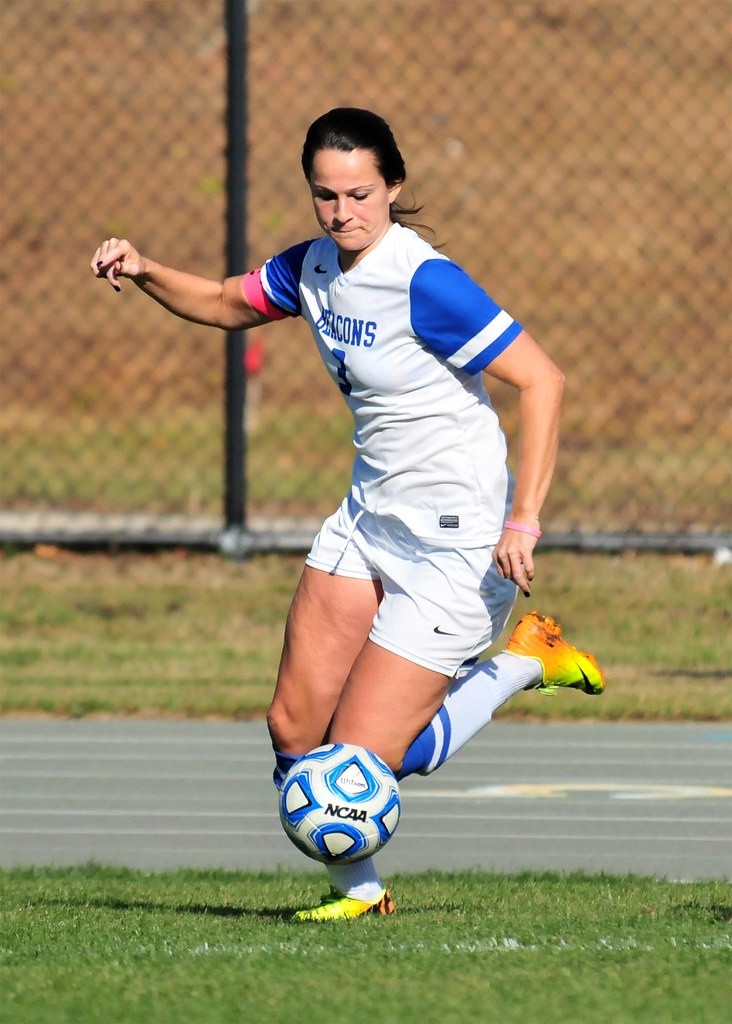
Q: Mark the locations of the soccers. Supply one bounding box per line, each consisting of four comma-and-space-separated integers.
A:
279, 741, 403, 867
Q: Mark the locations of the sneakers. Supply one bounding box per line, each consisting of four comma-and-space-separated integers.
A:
290, 884, 397, 923
505, 610, 607, 697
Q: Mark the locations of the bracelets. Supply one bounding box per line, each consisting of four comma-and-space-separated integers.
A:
504, 520, 542, 538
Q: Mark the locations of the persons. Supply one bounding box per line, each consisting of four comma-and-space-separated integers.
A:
90, 108, 606, 923
